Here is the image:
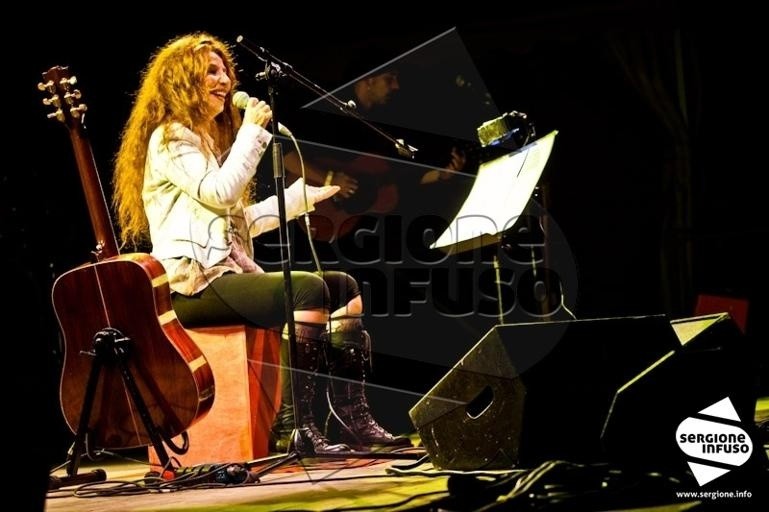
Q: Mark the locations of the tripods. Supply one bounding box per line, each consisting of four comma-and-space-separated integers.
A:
242, 83, 429, 480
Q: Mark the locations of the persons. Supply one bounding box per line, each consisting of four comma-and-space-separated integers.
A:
277, 48, 467, 198
115, 33, 415, 457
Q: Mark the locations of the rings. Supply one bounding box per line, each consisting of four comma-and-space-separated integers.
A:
350, 188, 354, 194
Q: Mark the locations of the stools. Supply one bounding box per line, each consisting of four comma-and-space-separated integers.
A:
148, 324, 282, 480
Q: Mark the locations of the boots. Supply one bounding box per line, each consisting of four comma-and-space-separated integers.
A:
322, 316, 415, 451
267, 318, 352, 459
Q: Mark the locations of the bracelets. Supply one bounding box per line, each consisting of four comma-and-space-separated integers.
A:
323, 169, 333, 186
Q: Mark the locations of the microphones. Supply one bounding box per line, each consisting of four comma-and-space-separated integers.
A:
232, 92, 292, 137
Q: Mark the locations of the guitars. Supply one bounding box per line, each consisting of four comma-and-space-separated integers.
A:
282, 112, 529, 242
38, 66, 214, 454
